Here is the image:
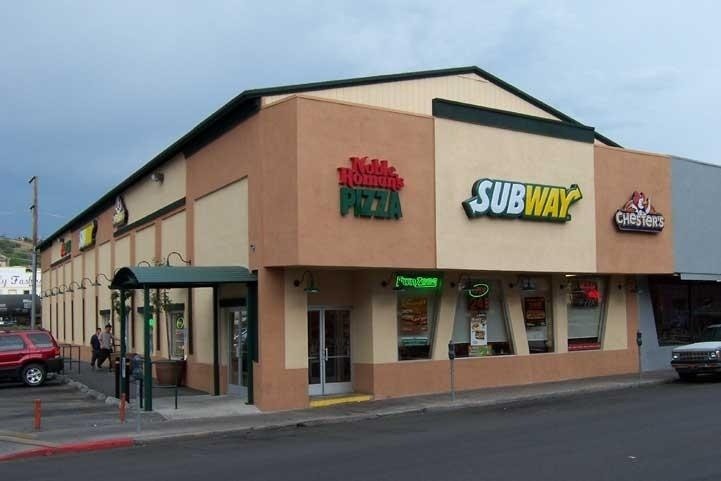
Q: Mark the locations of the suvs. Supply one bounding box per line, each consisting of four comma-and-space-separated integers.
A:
0, 326, 64, 387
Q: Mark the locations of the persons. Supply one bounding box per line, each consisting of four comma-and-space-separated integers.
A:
98, 324, 118, 372
88, 328, 102, 370
662, 314, 712, 341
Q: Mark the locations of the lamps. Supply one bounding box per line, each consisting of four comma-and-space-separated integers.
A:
294, 270, 320, 294
508, 274, 537, 292
450, 271, 478, 291
383, 271, 409, 292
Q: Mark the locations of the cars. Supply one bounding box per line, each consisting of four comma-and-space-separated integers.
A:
670, 324, 721, 382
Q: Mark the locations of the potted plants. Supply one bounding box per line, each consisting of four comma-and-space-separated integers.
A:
108, 289, 187, 386
